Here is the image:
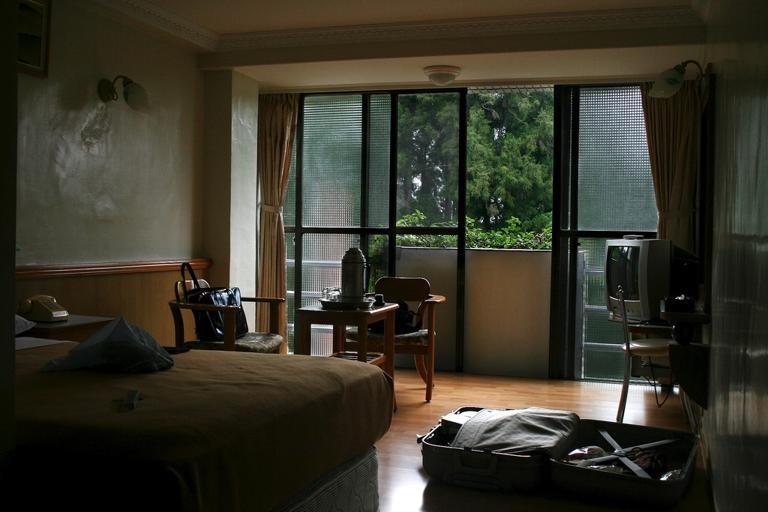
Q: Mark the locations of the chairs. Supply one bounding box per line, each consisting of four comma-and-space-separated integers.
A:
615, 284, 702, 428
169, 278, 288, 355
343, 275, 447, 403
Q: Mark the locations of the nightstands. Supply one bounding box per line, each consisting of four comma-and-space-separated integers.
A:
22, 312, 117, 343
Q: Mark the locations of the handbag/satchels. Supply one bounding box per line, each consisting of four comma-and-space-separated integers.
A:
184, 287, 248, 339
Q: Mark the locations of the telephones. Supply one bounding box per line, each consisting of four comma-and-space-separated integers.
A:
17, 294, 69, 322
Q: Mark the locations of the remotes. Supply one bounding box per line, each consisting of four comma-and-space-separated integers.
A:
124, 389, 141, 409
622, 234, 644, 238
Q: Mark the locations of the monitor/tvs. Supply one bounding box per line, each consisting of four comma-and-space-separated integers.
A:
603, 238, 701, 324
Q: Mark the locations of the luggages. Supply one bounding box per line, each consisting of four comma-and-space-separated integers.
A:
417, 407, 699, 501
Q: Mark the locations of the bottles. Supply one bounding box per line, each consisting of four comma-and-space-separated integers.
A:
341, 247, 371, 302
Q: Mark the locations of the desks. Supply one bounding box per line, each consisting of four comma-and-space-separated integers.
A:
294, 301, 401, 413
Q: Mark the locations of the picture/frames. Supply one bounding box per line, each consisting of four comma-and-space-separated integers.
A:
18, 0, 54, 80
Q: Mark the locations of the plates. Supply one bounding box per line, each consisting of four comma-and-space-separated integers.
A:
317, 298, 377, 308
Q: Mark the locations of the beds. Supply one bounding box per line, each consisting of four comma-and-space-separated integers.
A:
12, 334, 396, 512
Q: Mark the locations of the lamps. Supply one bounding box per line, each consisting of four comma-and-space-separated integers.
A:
423, 65, 462, 87
97, 73, 148, 111
